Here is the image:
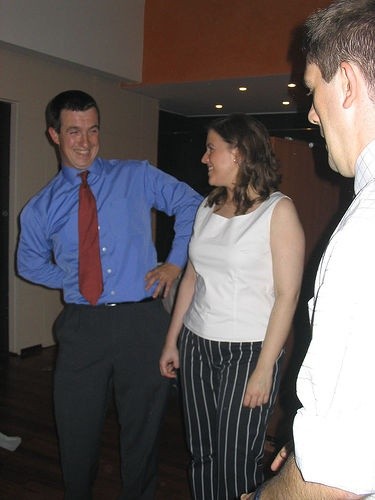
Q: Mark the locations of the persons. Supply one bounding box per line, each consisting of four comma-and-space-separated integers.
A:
238, 0, 375, 500
14, 90, 205, 500
158, 111, 306, 500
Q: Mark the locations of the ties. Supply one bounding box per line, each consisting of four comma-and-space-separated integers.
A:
78, 170, 104, 306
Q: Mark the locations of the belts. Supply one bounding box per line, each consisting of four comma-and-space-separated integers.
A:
102, 295, 159, 308
